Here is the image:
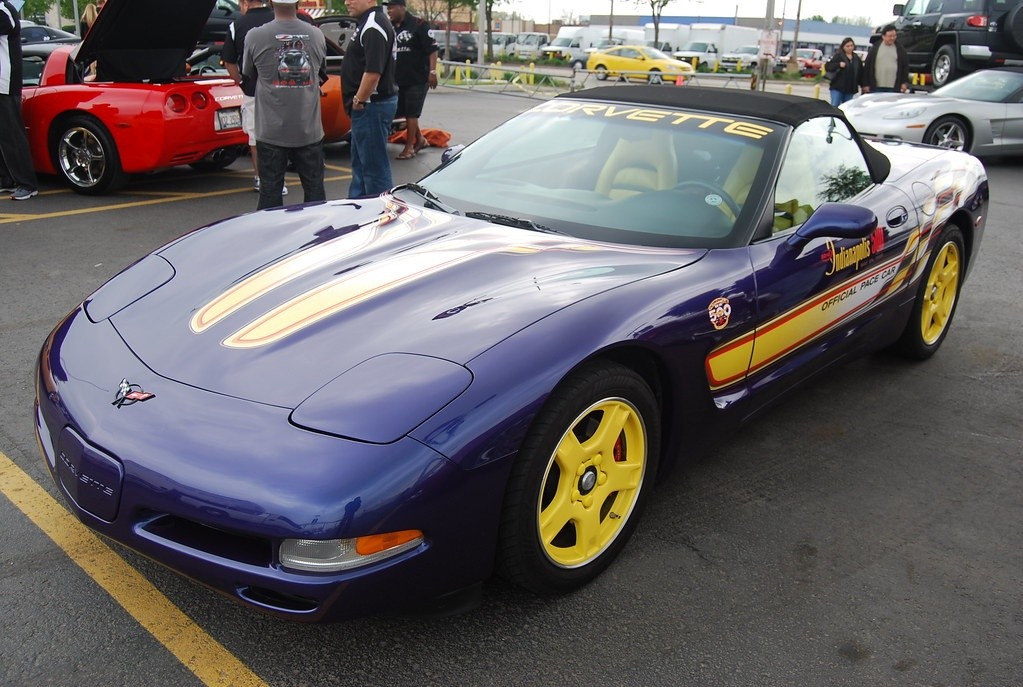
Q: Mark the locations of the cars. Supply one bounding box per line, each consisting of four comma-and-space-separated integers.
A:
870, 1, 1022, 84
838, 65, 1023, 158
30, 81, 993, 629
0, 1, 249, 190
587, 44, 696, 85
430, 24, 870, 75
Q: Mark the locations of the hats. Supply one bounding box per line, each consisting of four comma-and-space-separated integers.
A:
382, 0, 405, 6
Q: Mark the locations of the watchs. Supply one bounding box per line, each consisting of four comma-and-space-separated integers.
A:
352, 96, 364, 105
430, 70, 437, 75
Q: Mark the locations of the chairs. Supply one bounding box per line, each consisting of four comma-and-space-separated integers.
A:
716, 142, 819, 231
595, 131, 678, 205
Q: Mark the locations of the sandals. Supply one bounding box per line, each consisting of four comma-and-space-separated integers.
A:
395, 138, 430, 159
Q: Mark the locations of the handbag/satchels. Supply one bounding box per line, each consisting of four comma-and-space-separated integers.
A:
823, 54, 843, 80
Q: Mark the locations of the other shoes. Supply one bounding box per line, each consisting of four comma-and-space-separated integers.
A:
254, 176, 288, 196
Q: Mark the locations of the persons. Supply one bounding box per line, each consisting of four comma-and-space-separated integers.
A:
339, 0, 400, 199
80, 0, 107, 76
825, 38, 868, 107
382, 0, 438, 159
0, 0, 38, 201
242, 0, 331, 211
864, 25, 908, 95
221, 0, 317, 195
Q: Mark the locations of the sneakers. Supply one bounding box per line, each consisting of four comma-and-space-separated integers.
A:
0, 183, 39, 200
